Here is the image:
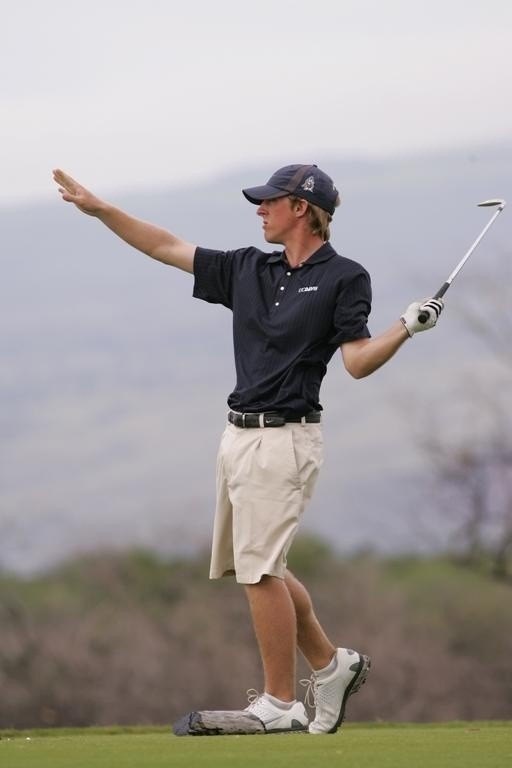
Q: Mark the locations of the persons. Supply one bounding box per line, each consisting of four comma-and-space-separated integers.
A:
48, 162, 447, 736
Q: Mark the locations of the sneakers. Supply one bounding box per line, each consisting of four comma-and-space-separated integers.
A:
299, 647, 371, 734
242, 688, 310, 732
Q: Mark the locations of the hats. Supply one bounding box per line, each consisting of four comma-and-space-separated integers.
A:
242, 164, 339, 215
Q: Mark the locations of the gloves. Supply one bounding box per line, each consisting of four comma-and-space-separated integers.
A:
399, 296, 444, 338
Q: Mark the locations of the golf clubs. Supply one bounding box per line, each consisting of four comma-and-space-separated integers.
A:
417, 198, 506, 323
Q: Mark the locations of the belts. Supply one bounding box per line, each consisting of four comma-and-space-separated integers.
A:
227, 412, 320, 428
